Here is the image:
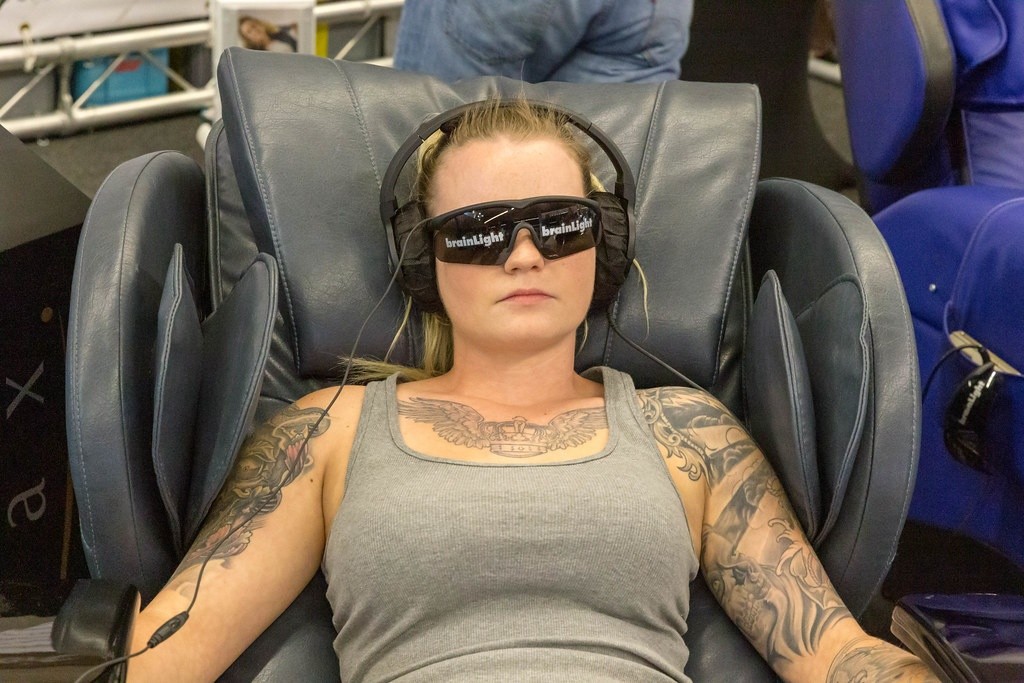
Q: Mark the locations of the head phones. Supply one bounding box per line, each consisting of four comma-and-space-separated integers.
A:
378, 99, 637, 316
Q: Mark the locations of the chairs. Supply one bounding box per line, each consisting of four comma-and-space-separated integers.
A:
828, 2, 1023, 681
63, 46, 922, 681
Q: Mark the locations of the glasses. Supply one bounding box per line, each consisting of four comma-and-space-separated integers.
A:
422, 195, 603, 266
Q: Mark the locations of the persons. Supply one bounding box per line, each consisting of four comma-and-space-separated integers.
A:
237, 15, 297, 53
392, 0, 694, 83
125, 90, 942, 682
